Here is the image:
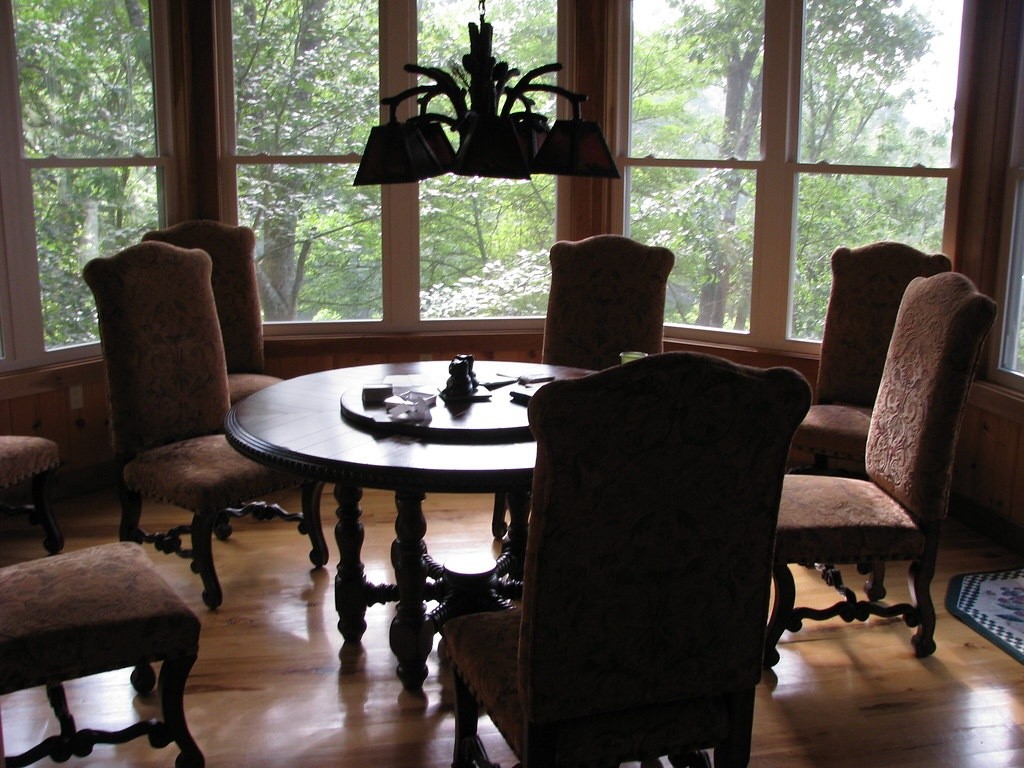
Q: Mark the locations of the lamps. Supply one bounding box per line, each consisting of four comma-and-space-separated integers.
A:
353, 1, 621, 186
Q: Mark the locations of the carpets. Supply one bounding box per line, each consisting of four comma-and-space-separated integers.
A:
945, 568, 1024, 665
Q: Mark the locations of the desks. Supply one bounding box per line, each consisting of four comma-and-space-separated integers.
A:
225, 362, 596, 690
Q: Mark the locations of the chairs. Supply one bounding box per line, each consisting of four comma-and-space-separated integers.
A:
443, 350, 814, 768
0, 436, 64, 552
761, 274, 999, 669
491, 235, 679, 538
0, 542, 205, 768
786, 241, 952, 602
142, 219, 285, 403
83, 242, 328, 609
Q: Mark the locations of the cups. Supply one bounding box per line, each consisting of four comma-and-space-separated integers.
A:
619, 351, 649, 365
